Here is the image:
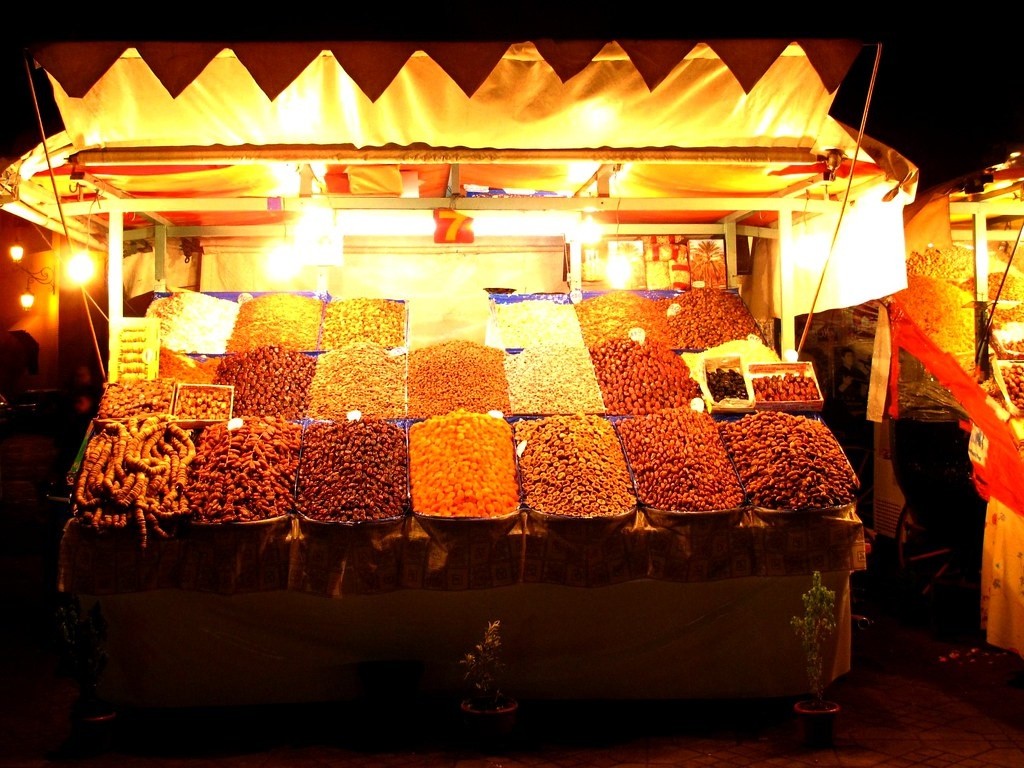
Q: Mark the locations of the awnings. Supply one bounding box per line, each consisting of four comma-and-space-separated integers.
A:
25, 39, 883, 376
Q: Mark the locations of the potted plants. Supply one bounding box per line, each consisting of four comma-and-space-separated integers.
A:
457, 620, 518, 748
788, 570, 840, 749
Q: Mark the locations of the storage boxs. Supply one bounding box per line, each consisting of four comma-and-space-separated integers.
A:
174, 383, 235, 426
750, 361, 824, 412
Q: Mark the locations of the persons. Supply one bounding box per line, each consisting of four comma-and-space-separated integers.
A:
836, 349, 867, 406
60, 366, 101, 447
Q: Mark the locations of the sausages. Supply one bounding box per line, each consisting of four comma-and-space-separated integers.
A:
76, 416, 198, 549
